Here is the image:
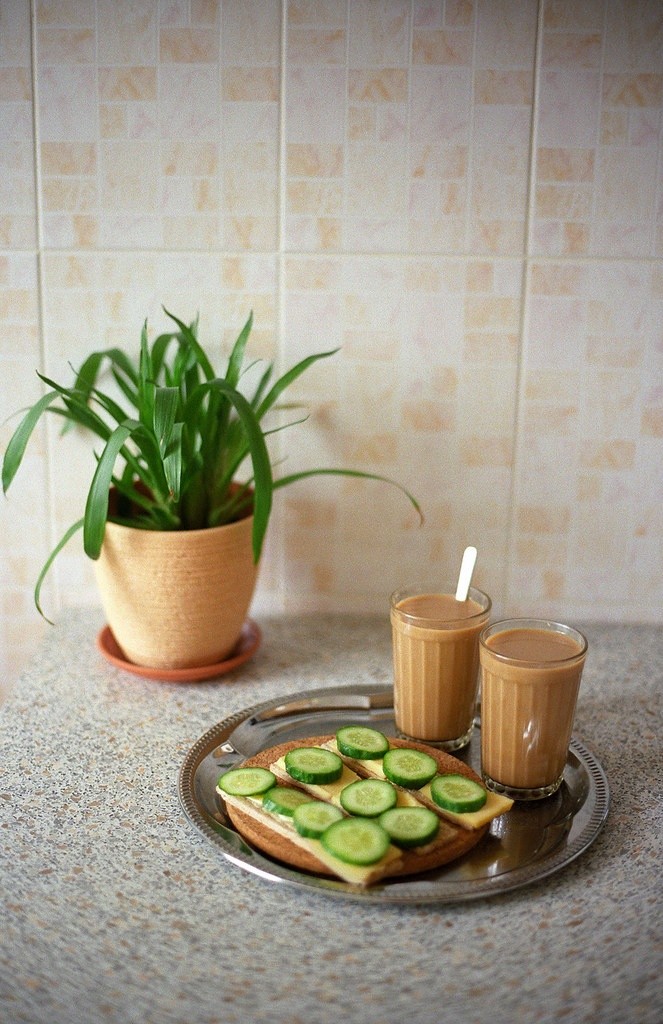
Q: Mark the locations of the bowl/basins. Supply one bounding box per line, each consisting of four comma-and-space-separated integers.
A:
97, 613, 263, 681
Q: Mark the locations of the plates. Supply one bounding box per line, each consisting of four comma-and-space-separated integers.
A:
175, 684, 612, 906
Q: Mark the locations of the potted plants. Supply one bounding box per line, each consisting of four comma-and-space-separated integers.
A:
0, 302, 424, 667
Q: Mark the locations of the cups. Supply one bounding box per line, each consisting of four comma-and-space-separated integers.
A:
477, 617, 589, 802
387, 578, 492, 753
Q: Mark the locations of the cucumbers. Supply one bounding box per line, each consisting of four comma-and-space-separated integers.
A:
218, 726, 487, 868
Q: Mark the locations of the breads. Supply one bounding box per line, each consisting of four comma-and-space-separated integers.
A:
216, 738, 514, 887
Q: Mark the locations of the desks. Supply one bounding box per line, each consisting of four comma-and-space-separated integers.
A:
0, 603, 663, 1024
